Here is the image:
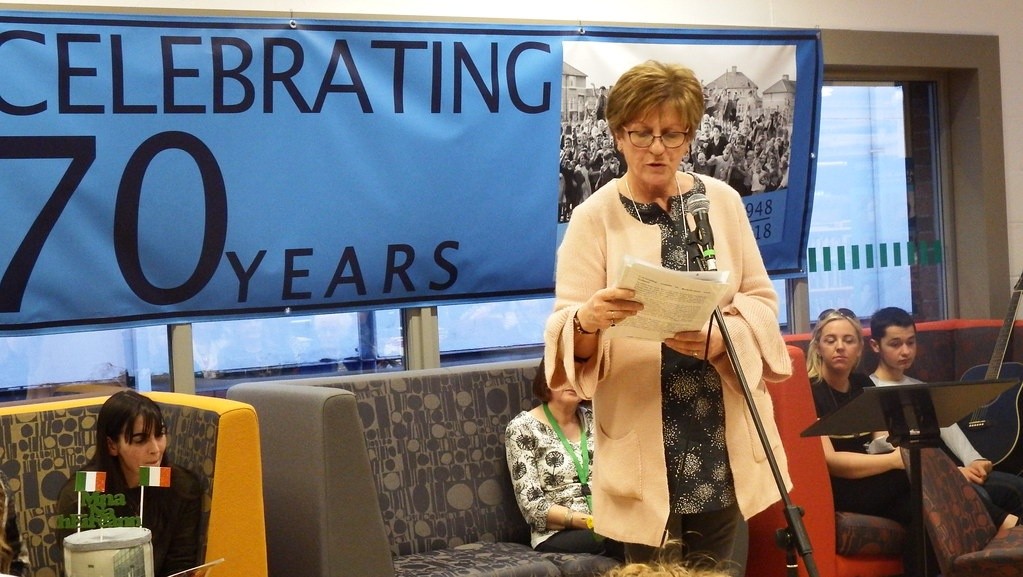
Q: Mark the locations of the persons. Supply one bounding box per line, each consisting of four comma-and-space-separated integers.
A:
54, 390, 203, 577
804, 306, 1023, 577
557, 83, 626, 222
678, 78, 790, 195
542, 61, 792, 577
504, 355, 624, 559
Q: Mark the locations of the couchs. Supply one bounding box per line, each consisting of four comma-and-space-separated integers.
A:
0, 391, 268, 577
749, 318, 1023, 577
226, 357, 619, 577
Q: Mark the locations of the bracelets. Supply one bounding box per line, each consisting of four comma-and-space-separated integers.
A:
574, 313, 600, 335
564, 509, 573, 529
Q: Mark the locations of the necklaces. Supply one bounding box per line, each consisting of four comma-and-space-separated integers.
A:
625, 174, 690, 273
829, 384, 860, 439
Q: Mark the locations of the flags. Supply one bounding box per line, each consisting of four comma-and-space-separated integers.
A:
75, 471, 106, 492
139, 466, 171, 487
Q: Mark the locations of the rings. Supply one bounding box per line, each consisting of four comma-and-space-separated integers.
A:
610, 320, 615, 326
612, 312, 615, 319
694, 351, 698, 358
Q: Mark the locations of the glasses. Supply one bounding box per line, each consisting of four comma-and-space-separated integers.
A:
817, 308, 856, 323
620, 124, 691, 149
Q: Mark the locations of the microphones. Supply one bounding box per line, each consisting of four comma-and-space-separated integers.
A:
686, 193, 717, 273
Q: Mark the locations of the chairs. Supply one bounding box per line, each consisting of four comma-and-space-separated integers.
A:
901, 440, 1023, 577
